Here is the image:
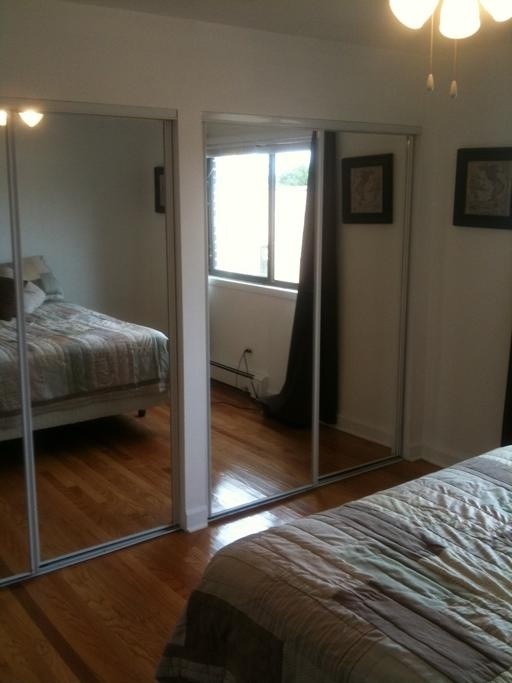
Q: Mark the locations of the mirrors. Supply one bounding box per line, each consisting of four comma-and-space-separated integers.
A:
0, 102, 180, 586
200, 109, 416, 520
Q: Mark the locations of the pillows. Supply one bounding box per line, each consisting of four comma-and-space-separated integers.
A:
0, 256, 62, 322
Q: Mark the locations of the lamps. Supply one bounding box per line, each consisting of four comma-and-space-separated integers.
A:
1, 109, 43, 128
385, 0, 511, 97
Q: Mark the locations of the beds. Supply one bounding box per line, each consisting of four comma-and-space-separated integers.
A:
0, 255, 171, 442
163, 445, 510, 680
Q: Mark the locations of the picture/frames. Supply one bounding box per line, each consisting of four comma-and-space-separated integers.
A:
342, 152, 394, 224
154, 166, 165, 212
453, 145, 512, 230
